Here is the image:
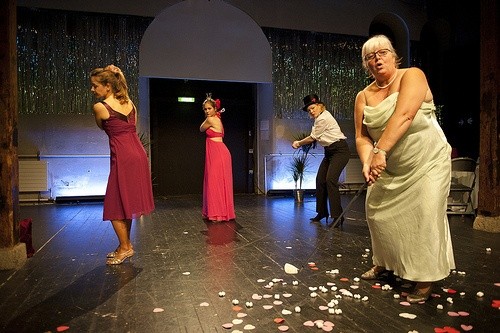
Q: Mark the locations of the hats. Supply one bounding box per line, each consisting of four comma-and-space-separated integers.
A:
303, 94, 324, 112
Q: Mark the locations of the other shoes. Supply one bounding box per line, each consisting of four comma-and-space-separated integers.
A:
406, 285, 432, 303
361, 265, 385, 279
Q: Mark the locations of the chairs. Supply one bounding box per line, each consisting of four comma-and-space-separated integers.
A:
447, 157, 476, 218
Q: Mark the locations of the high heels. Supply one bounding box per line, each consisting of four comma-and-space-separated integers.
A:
310, 213, 329, 221
330, 215, 344, 228
106, 249, 134, 265
106, 241, 133, 257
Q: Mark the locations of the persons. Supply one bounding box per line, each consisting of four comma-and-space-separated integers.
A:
90, 64, 155, 265
291, 94, 350, 228
354, 35, 457, 302
199, 97, 237, 223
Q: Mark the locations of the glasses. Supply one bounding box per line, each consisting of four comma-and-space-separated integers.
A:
365, 49, 393, 60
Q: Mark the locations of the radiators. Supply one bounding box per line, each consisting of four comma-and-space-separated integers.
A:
344, 158, 366, 184
17, 160, 48, 192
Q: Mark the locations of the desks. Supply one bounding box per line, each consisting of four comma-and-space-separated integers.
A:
448, 165, 479, 213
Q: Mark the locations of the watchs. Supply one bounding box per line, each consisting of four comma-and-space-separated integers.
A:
373, 148, 387, 154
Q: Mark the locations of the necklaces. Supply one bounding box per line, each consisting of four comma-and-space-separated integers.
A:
105, 92, 112, 98
376, 69, 397, 89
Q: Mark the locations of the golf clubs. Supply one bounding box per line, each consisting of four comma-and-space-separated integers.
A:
284, 178, 370, 274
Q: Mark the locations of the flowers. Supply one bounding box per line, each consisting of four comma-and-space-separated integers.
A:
214, 98, 221, 109
312, 98, 316, 101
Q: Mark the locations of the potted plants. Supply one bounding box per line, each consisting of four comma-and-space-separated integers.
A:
286, 131, 317, 203
